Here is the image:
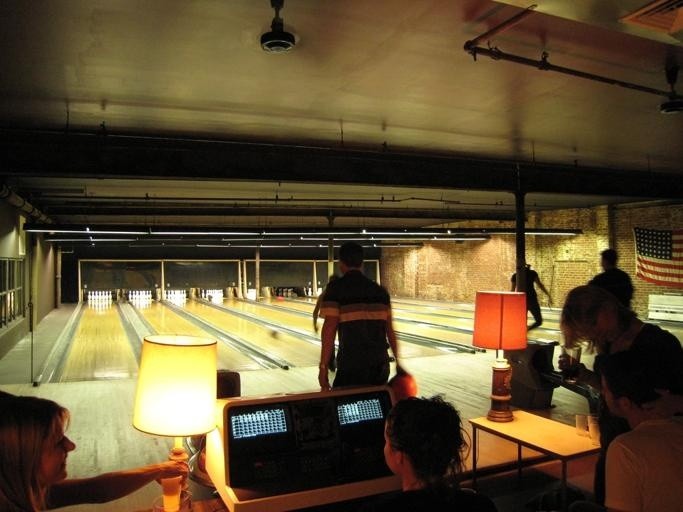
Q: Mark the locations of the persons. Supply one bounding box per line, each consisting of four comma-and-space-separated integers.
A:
312, 275, 340, 372
381, 392, 499, 511
569, 321, 682, 512
0, 393, 190, 511
510, 265, 556, 331
556, 284, 682, 504
318, 241, 405, 392
593, 248, 632, 309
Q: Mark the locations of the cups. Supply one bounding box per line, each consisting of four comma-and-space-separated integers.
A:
562, 345, 582, 384
160, 476, 182, 512
575, 414, 588, 436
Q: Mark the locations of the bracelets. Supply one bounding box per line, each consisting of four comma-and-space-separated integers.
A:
319, 363, 329, 369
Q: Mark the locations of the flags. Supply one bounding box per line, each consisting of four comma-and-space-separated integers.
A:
633, 227, 683, 289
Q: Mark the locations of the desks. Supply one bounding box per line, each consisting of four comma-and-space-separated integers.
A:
469, 410, 602, 512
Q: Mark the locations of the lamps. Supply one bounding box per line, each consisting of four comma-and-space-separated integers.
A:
259, 0, 295, 53
130, 333, 220, 512
472, 290, 528, 424
